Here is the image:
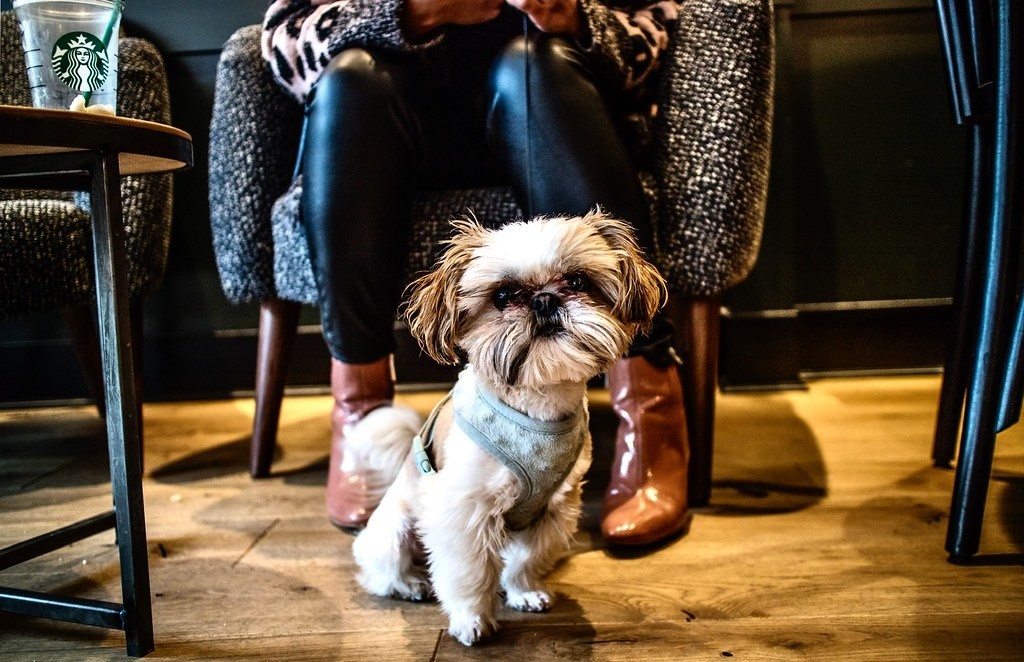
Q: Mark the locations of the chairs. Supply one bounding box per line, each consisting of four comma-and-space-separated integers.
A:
206, 0, 777, 510
924, 1, 1024, 563
0, 8, 177, 507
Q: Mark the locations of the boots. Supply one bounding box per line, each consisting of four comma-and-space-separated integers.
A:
328, 353, 396, 526
598, 355, 691, 542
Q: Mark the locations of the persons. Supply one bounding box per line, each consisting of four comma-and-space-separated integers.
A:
256, 0, 692, 547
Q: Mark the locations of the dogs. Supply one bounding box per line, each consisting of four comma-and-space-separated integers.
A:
341, 203, 669, 648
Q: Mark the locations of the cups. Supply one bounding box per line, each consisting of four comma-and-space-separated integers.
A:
10, 0, 126, 116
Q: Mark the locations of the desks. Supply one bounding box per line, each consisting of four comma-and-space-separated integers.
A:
0, 100, 195, 655
